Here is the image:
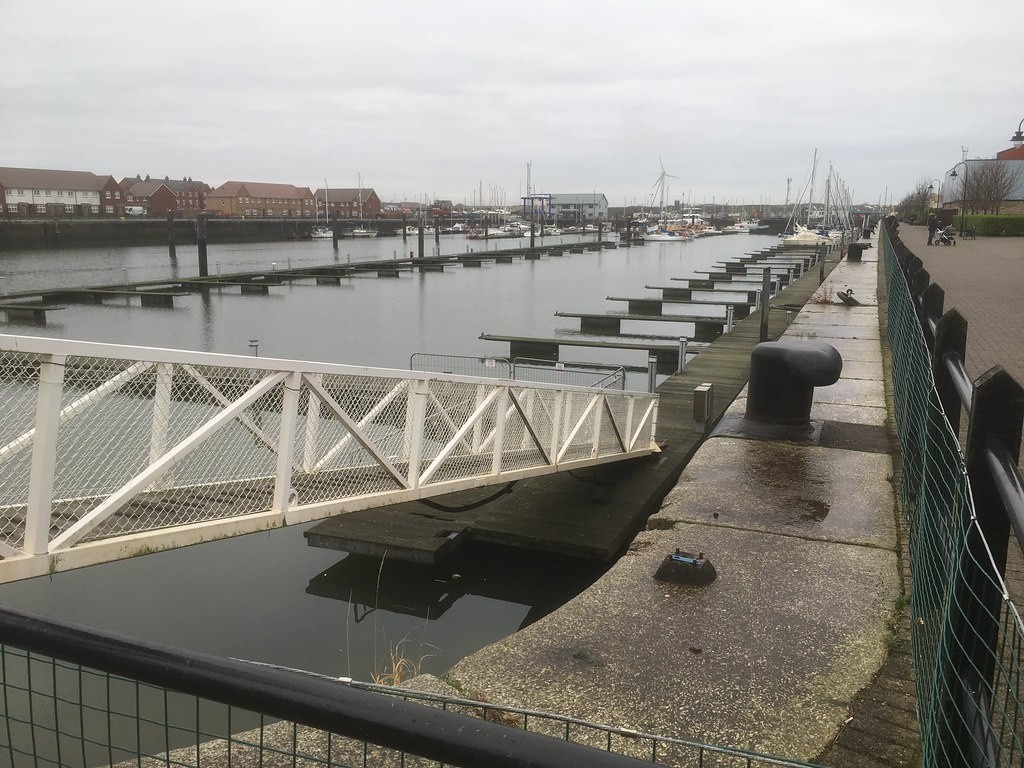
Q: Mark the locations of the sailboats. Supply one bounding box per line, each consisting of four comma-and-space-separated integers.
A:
776, 148, 861, 245
345, 171, 379, 240
641, 165, 763, 237
311, 179, 334, 237
400, 180, 603, 240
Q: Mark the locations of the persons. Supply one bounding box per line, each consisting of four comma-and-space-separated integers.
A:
927, 213, 942, 245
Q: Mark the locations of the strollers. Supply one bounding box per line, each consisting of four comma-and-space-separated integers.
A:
934, 225, 957, 246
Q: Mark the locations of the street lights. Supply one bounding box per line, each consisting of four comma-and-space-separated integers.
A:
928, 178, 941, 214
950, 162, 968, 237
